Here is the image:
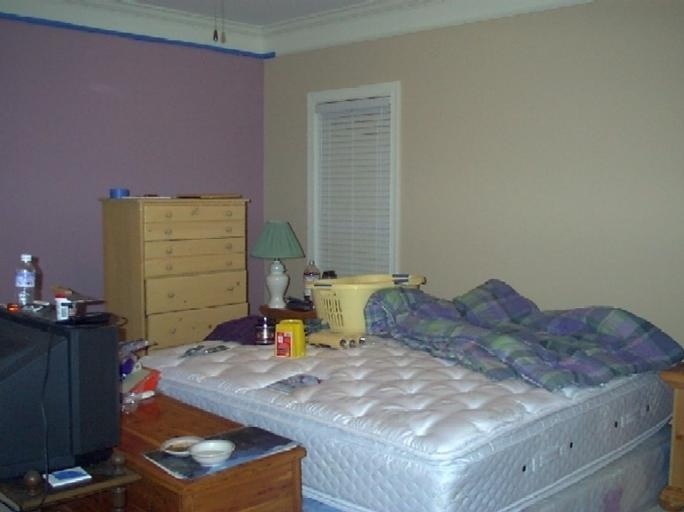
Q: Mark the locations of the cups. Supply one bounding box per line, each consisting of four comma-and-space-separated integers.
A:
54, 297, 72, 322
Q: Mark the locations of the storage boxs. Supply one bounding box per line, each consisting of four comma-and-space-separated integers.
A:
308, 273, 425, 338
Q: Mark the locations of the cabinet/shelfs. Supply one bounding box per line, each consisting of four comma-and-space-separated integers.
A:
95, 195, 247, 359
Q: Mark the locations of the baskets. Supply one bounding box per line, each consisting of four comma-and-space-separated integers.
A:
302, 270, 428, 343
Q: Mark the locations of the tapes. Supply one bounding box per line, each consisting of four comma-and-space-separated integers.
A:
109, 188, 129, 199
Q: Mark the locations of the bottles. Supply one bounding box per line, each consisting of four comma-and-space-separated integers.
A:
13, 253, 38, 310
302, 259, 321, 302
253, 316, 275, 345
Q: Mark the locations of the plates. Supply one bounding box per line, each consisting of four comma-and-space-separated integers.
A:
160, 436, 206, 459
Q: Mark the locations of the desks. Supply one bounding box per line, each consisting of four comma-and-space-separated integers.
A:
116, 393, 308, 511
1, 457, 142, 511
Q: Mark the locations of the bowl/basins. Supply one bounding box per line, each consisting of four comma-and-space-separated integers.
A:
187, 439, 236, 468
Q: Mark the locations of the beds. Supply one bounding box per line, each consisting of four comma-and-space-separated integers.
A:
134, 332, 672, 512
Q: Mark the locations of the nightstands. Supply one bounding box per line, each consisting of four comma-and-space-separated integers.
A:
658, 363, 683, 511
256, 301, 315, 324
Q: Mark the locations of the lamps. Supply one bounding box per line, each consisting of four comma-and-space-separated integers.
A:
249, 221, 305, 310
